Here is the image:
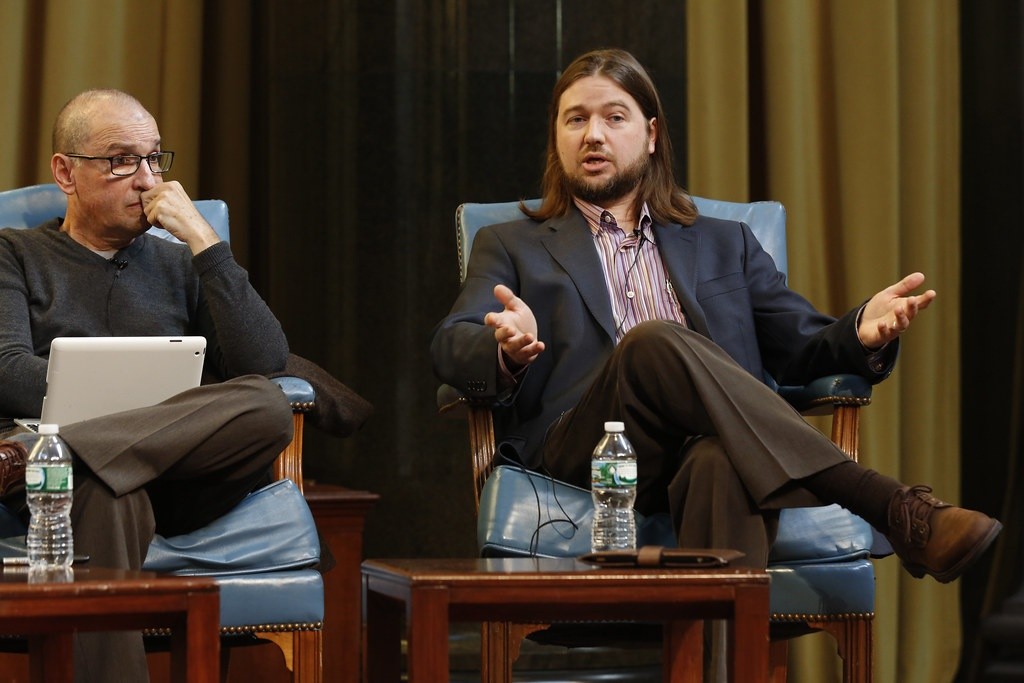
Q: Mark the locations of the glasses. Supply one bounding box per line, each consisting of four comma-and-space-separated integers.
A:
65, 150, 174, 177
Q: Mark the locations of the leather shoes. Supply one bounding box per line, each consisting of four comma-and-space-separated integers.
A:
888, 485, 1002, 583
0, 440, 29, 498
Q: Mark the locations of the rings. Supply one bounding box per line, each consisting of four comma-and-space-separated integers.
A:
892, 322, 907, 333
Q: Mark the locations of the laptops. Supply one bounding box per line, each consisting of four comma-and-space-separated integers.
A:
14, 335, 207, 436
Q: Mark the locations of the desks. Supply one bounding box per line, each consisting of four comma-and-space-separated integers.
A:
0, 567, 220, 683
306, 482, 380, 683
358, 552, 773, 683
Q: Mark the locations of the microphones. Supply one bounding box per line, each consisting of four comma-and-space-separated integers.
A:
638, 233, 649, 251
117, 260, 128, 272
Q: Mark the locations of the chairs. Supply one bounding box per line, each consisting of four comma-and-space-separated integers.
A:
447, 185, 878, 683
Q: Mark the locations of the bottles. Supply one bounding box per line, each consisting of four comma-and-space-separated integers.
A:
592, 421, 636, 552
26, 424, 73, 583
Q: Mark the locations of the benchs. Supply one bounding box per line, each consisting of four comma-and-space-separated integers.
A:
0, 180, 324, 683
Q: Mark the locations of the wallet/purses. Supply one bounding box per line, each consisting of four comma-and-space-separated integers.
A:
579, 545, 746, 568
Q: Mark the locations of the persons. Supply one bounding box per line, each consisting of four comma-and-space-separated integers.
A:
0, 89, 295, 683
424, 49, 1003, 583
